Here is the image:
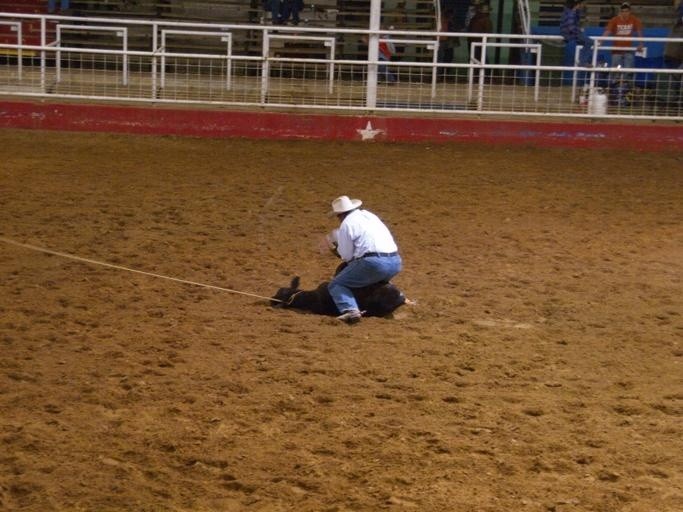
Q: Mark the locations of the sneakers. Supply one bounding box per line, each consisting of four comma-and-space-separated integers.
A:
336, 310, 362, 324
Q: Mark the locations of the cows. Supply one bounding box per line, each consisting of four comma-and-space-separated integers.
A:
269, 261, 416, 317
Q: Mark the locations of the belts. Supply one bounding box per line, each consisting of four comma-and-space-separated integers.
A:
364, 252, 398, 257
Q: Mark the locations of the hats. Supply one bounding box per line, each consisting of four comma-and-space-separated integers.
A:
327, 195, 363, 217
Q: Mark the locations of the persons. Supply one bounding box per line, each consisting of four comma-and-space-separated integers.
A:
270, 1, 285, 33
431, 2, 459, 82
465, 3, 493, 83
389, 0, 407, 61
323, 195, 402, 324
359, 23, 398, 87
279, 0, 305, 26
559, 1, 594, 69
590, 2, 644, 107
